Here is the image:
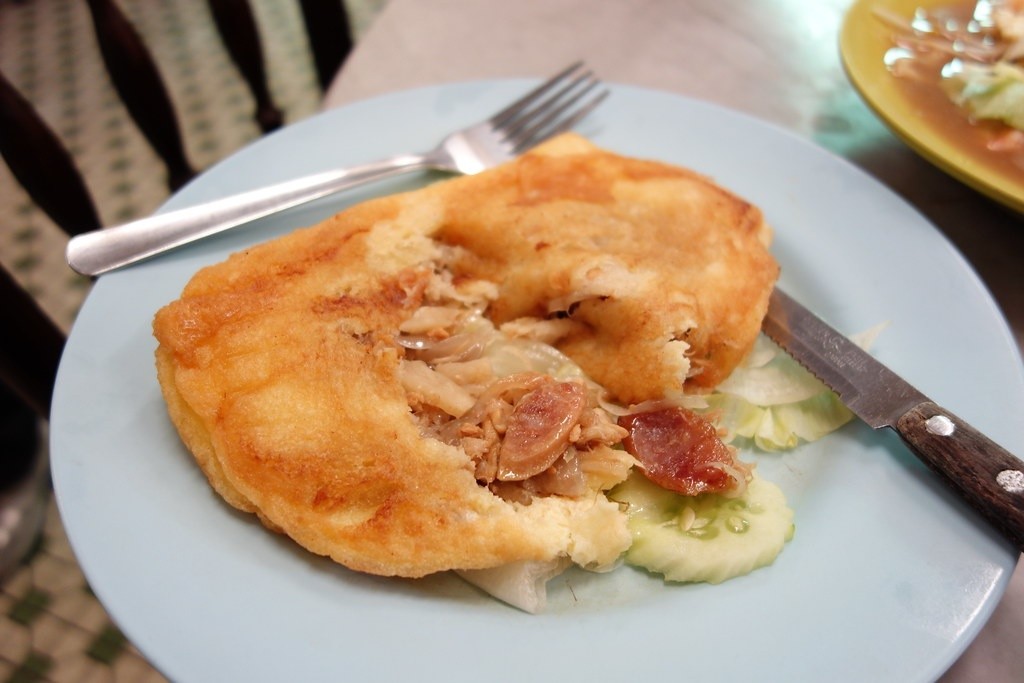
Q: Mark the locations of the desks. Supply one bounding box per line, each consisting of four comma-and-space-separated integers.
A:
327, 1, 1024, 683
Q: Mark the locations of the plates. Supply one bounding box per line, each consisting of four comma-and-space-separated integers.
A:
49, 77, 1024, 683
837, 0, 1024, 211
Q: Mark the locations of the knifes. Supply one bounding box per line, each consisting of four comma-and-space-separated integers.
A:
761, 286, 1024, 554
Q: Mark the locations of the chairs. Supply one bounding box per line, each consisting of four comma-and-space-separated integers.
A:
0, 0, 351, 428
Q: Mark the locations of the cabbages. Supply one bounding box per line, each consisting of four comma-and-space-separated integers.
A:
706, 315, 886, 451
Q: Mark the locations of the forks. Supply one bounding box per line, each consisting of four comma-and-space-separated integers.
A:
64, 60, 611, 278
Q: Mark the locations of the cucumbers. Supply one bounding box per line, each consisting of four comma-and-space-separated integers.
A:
602, 459, 795, 582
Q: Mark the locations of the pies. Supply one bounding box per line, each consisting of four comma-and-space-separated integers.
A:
152, 130, 782, 580
